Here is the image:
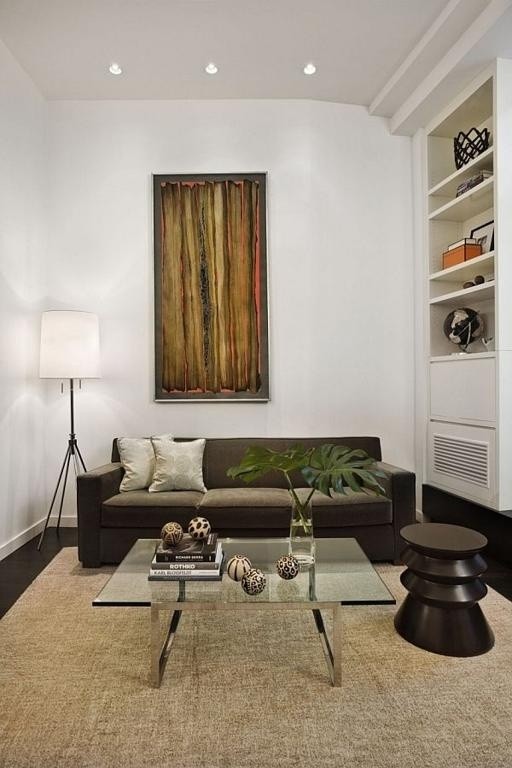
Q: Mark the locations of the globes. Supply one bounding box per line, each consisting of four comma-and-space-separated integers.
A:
443, 305, 484, 355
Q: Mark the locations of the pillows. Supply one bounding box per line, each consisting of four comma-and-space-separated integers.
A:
115, 435, 211, 496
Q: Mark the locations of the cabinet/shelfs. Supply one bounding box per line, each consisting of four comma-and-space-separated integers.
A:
420, 60, 497, 366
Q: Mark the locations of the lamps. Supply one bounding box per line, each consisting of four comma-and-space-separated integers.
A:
31, 310, 101, 553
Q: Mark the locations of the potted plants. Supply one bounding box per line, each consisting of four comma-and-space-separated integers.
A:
227, 440, 393, 566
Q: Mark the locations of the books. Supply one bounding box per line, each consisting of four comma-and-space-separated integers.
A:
148, 533, 227, 581
456, 170, 493, 197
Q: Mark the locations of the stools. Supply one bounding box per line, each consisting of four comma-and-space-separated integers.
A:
393, 520, 497, 655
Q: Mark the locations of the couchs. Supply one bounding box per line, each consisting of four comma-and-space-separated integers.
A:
76, 433, 416, 566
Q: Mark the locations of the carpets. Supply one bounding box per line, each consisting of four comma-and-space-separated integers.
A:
0, 545, 512, 767
